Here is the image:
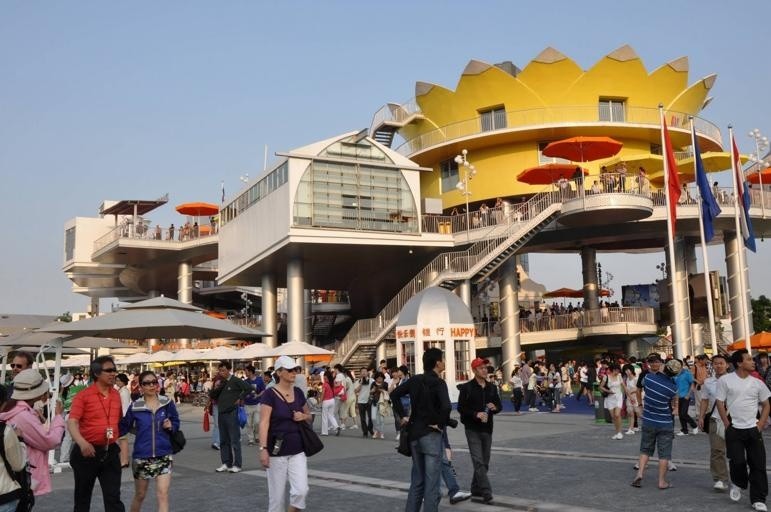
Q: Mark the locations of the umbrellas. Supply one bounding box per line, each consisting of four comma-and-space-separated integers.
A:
575, 286, 613, 297
746, 165, 770, 184
543, 286, 580, 305
727, 330, 769, 352
542, 136, 622, 178
515, 163, 587, 190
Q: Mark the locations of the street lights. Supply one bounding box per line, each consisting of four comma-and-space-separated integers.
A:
601, 270, 615, 304
656, 261, 667, 279
454, 148, 475, 240
748, 127, 769, 218
239, 172, 250, 187
240, 291, 252, 326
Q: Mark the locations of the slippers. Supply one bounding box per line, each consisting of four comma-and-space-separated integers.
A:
658, 482, 672, 489
629, 481, 641, 487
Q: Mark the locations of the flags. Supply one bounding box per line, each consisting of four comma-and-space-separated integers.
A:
220, 186, 225, 202
121, 217, 219, 245
663, 116, 679, 245
730, 135, 755, 256
691, 124, 721, 244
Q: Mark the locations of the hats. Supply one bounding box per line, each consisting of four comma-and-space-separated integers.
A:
471, 357, 490, 368
646, 353, 661, 362
59, 375, 74, 387
9, 369, 50, 401
271, 356, 301, 375
665, 359, 681, 374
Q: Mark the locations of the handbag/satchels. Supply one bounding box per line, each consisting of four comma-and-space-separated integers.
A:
702, 401, 716, 433
600, 375, 609, 398
203, 409, 210, 432
272, 386, 323, 456
168, 426, 186, 454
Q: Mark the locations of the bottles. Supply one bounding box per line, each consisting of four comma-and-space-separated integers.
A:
482, 404, 490, 423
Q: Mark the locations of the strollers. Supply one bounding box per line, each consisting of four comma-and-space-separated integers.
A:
537, 387, 555, 407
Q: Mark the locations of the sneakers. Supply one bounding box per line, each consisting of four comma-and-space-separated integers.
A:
371, 432, 378, 439
633, 461, 648, 469
729, 484, 742, 501
668, 461, 677, 471
752, 501, 768, 511
320, 424, 358, 436
450, 491, 472, 505
677, 432, 688, 435
611, 426, 640, 440
380, 433, 385, 439
713, 481, 728, 490
693, 427, 698, 434
211, 442, 242, 473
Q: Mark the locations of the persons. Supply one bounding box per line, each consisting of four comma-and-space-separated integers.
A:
1, 348, 413, 452
631, 353, 682, 489
256, 357, 313, 511
389, 349, 503, 510
698, 352, 771, 511
207, 362, 253, 473
468, 299, 638, 334
68, 355, 180, 511
446, 165, 763, 234
174, 201, 220, 215
510, 351, 771, 439
0, 349, 66, 511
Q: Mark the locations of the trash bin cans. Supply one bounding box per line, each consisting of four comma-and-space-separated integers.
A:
119, 437, 129, 467
593, 382, 605, 422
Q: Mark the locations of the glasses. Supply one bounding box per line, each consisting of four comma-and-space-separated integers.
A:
285, 369, 298, 373
142, 380, 158, 386
10, 362, 23, 369
102, 368, 117, 373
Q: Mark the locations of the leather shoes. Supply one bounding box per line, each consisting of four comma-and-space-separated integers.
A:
483, 493, 492, 501
472, 493, 482, 497
